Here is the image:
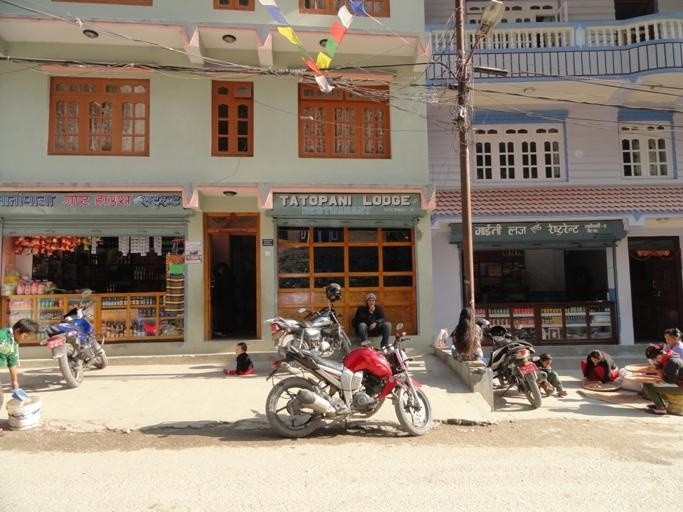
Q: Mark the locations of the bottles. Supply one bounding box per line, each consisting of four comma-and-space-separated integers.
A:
17, 278, 57, 294
103, 296, 156, 339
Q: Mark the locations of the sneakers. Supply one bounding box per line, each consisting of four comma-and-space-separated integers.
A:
545, 387, 569, 398
650, 405, 668, 415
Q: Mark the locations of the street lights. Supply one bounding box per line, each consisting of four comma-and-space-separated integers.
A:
458, 0, 506, 307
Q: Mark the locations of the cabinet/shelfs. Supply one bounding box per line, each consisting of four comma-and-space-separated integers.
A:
8, 291, 184, 345
474, 301, 619, 345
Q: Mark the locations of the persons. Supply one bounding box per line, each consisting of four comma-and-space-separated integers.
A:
352, 292, 392, 347
664, 328, 683, 360
536, 353, 567, 396
0, 316, 43, 433
642, 345, 683, 414
581, 350, 619, 385
223, 342, 255, 376
451, 307, 484, 361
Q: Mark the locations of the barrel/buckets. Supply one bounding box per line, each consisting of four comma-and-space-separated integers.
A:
6, 397, 41, 431
667, 393, 683, 414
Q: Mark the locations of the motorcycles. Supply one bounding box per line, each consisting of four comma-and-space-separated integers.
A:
477, 318, 542, 408
263, 283, 352, 362
45, 289, 109, 388
266, 322, 431, 436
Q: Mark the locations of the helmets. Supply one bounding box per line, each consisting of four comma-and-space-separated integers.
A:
325, 283, 341, 297
364, 293, 376, 304
